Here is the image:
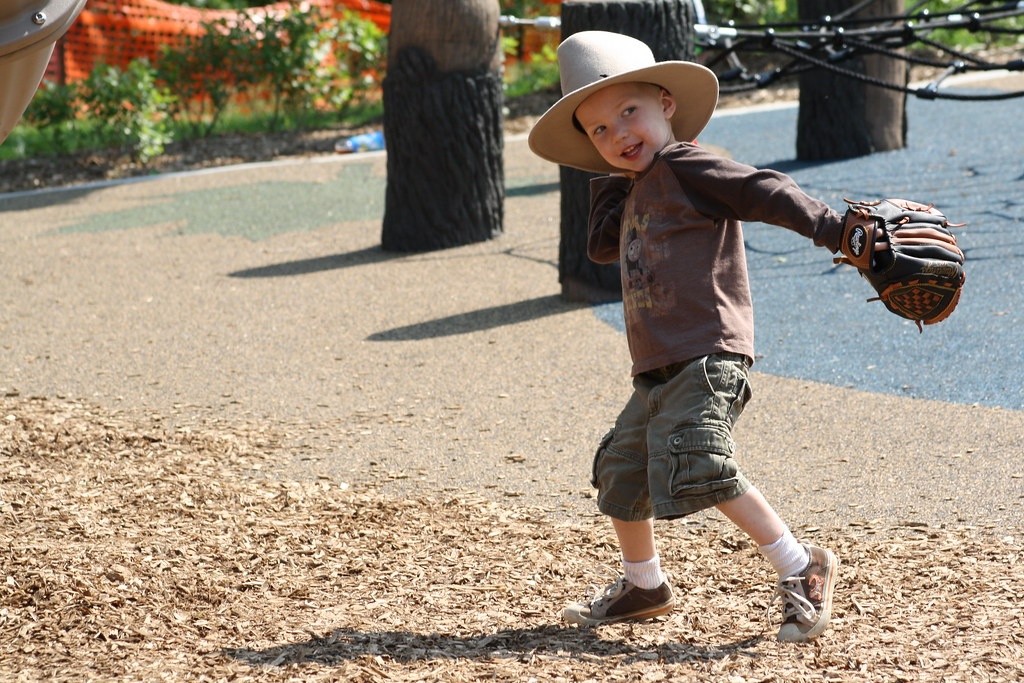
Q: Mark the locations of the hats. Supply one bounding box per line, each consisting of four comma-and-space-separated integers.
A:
527, 31, 720, 173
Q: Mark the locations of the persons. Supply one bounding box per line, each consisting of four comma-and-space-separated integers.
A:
528, 31, 965, 642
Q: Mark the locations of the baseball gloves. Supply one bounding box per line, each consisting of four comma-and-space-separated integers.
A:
832, 197, 966, 325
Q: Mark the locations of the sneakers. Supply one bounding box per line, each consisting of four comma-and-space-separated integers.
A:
767, 543, 837, 642
564, 566, 674, 627
